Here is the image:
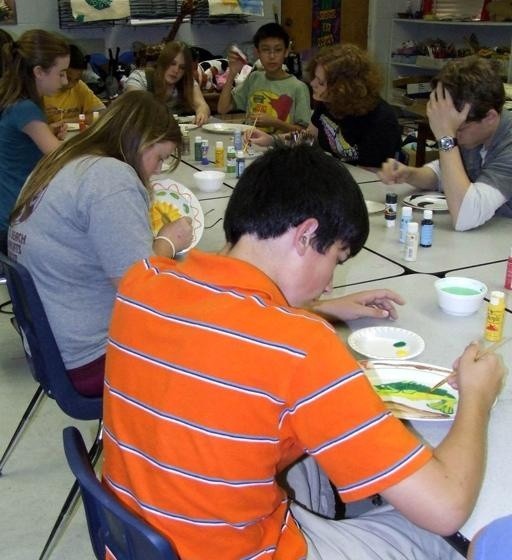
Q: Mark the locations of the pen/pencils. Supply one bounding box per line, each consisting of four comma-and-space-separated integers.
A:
61, 109, 64, 129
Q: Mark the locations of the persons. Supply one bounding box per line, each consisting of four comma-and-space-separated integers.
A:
124, 39, 212, 129
217, 22, 312, 133
379, 56, 512, 232
240, 43, 403, 178
1, 27, 72, 255
96, 136, 507, 560
38, 43, 107, 126
8, 90, 196, 398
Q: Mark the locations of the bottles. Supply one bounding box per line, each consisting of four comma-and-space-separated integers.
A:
79, 114, 87, 131
385, 193, 398, 228
402, 222, 418, 262
173, 114, 246, 179
92, 112, 99, 123
419, 210, 433, 247
399, 207, 413, 244
504, 248, 512, 290
485, 290, 506, 341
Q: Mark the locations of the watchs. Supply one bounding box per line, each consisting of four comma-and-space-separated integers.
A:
436, 137, 458, 152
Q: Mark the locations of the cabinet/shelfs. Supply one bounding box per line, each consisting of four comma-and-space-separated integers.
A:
387, 16, 511, 117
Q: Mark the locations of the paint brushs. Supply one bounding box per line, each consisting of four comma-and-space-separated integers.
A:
243, 106, 262, 153
429, 336, 512, 394
394, 151, 399, 185
272, 128, 316, 147
154, 206, 172, 223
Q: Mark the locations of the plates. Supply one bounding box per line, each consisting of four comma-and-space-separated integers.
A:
64, 122, 89, 131
404, 192, 449, 211
203, 123, 256, 135
357, 358, 497, 420
178, 124, 198, 131
147, 178, 205, 255
364, 200, 386, 214
348, 326, 424, 360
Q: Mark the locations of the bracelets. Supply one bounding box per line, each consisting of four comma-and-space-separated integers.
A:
154, 236, 176, 259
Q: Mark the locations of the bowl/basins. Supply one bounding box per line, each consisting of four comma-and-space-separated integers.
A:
193, 171, 225, 192
435, 277, 488, 316
